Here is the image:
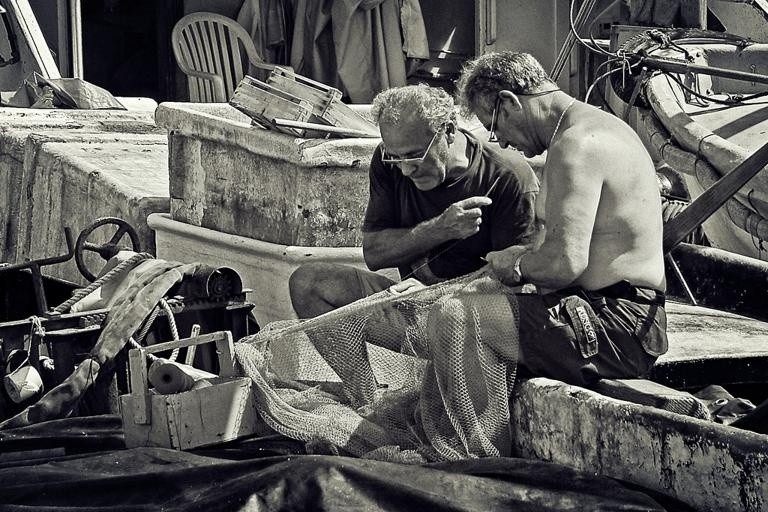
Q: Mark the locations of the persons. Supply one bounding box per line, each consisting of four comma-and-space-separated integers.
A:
289, 85, 548, 430
420, 50, 671, 458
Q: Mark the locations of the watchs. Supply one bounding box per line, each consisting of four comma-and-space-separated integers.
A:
512, 251, 529, 287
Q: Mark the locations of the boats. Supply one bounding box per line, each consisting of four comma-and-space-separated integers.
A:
631, 28, 768, 263
0, 215, 263, 449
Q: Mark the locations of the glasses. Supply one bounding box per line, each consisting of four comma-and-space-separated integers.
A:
488, 96, 502, 142
381, 131, 438, 165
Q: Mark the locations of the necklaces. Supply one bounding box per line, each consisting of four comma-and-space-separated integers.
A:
542, 95, 579, 151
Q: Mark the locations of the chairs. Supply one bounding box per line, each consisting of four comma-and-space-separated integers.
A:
170, 10, 296, 104
